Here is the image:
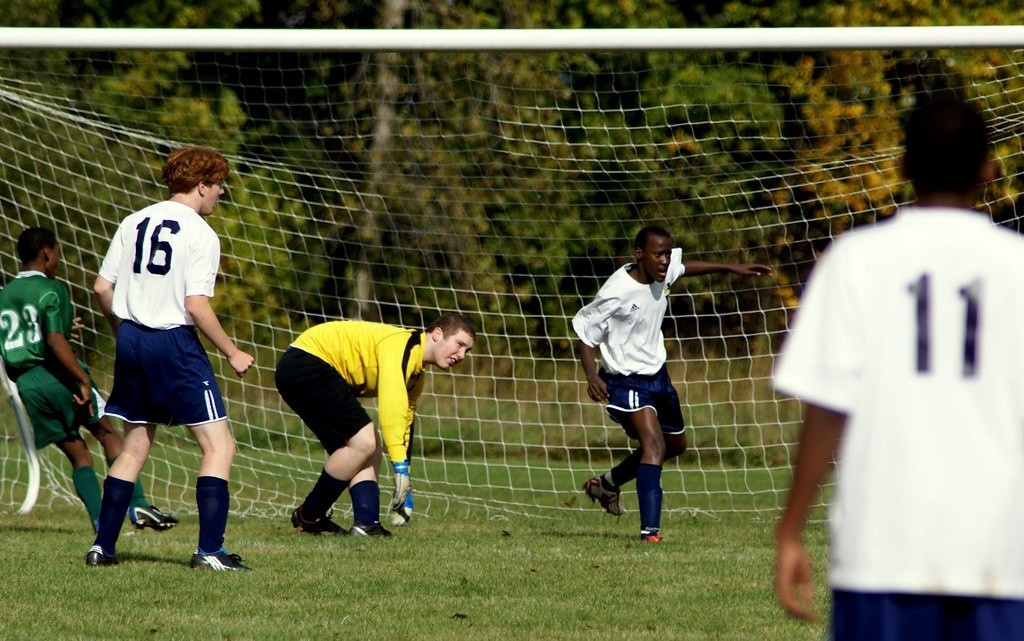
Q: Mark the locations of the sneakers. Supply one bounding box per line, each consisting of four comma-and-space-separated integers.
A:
291, 505, 348, 537
349, 522, 391, 538
583, 476, 624, 526
643, 534, 661, 543
128, 505, 179, 532
92, 517, 100, 536
191, 547, 253, 573
86, 544, 119, 566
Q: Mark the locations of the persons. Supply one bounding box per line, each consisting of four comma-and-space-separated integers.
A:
770, 92, 1023, 641
86, 148, 256, 572
0, 226, 178, 535
571, 226, 772, 545
275, 312, 475, 539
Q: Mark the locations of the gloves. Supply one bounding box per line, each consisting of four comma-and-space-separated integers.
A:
392, 490, 413, 527
392, 462, 411, 511
